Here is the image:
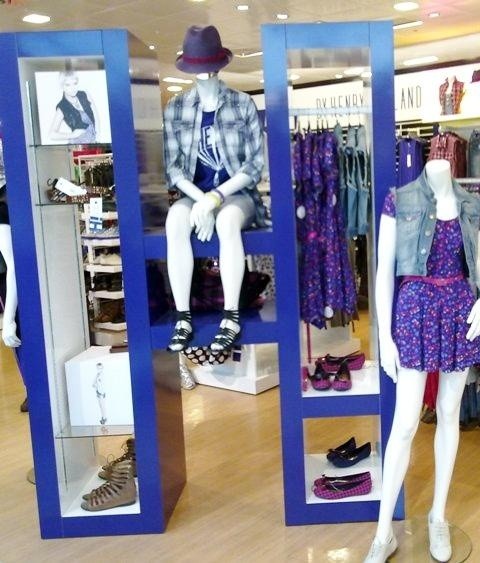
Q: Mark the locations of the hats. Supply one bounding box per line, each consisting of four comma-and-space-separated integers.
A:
175, 24, 233, 73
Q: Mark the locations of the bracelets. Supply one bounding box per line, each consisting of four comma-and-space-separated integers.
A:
205, 188, 225, 207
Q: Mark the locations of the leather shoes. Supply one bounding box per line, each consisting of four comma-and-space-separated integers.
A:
81, 223, 126, 323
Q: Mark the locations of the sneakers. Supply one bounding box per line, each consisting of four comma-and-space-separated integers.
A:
427, 513, 452, 562
363, 534, 397, 563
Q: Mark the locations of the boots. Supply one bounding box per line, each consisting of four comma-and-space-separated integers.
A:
80, 437, 136, 512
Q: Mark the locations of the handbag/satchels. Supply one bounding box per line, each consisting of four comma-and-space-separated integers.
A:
158, 256, 271, 314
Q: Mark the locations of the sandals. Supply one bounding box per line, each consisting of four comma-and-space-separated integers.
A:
326, 436, 372, 467
210, 309, 243, 351
313, 471, 372, 500
307, 350, 365, 391
167, 310, 194, 352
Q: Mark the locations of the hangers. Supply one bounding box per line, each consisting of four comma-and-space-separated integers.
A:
294, 106, 367, 153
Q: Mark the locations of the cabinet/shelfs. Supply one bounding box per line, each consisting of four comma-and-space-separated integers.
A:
0, 26, 185, 540
259, 20, 408, 528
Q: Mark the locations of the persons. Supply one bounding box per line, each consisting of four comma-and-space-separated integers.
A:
50, 71, 95, 144
364, 159, 480, 563
163, 26, 264, 353
0, 183, 29, 412
